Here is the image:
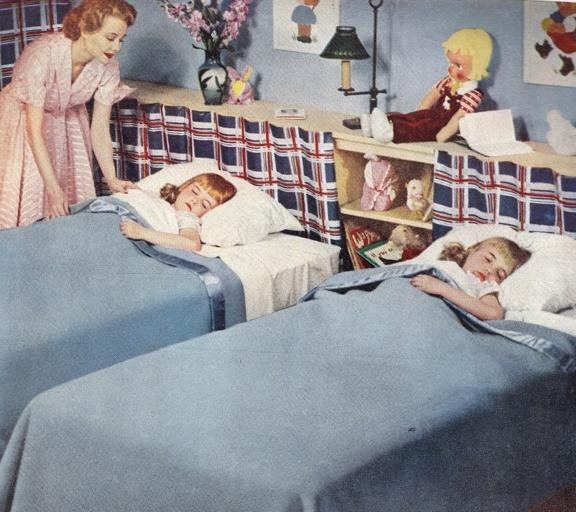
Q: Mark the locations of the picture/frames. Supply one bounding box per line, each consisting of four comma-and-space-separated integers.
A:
272, 0, 339, 55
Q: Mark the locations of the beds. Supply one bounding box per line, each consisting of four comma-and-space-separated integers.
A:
0, 158, 342, 461
0, 226, 576, 512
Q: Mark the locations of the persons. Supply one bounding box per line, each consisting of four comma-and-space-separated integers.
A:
0, 1, 139, 229
360, 28, 493, 143
410, 237, 531, 320
120, 174, 237, 252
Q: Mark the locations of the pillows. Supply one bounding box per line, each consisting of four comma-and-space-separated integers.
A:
133, 158, 304, 246
411, 224, 576, 314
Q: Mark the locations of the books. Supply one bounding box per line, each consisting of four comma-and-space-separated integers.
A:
276, 107, 306, 120
456, 109, 535, 157
345, 226, 398, 270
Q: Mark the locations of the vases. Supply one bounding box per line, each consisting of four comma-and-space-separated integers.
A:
198, 50, 227, 106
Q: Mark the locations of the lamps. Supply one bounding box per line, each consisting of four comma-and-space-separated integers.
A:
320, 0, 387, 131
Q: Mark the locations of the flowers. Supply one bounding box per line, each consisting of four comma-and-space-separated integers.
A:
157, 0, 255, 53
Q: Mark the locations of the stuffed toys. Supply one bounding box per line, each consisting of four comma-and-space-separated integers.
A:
389, 225, 427, 261
225, 65, 256, 106
361, 154, 429, 219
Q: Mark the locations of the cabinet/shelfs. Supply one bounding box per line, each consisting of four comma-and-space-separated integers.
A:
328, 132, 437, 271
